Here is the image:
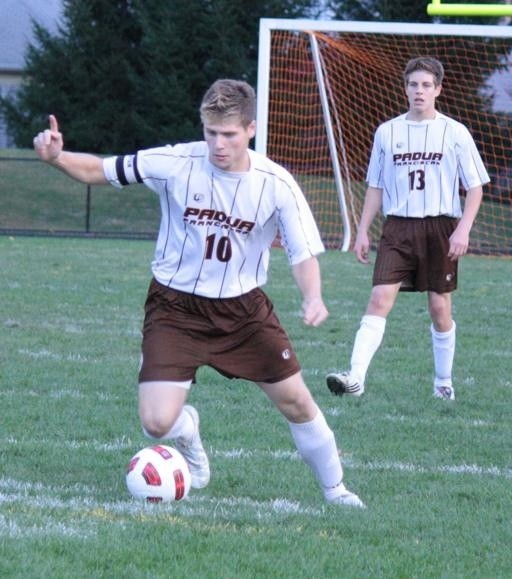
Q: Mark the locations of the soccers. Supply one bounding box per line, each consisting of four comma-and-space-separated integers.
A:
126, 444, 190, 503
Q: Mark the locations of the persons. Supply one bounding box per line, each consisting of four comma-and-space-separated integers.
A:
323, 56, 491, 401
33, 77, 365, 509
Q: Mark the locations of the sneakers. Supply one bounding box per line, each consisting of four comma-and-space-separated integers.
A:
175, 407, 209, 489
326, 371, 362, 397
432, 381, 455, 401
326, 489, 365, 512
